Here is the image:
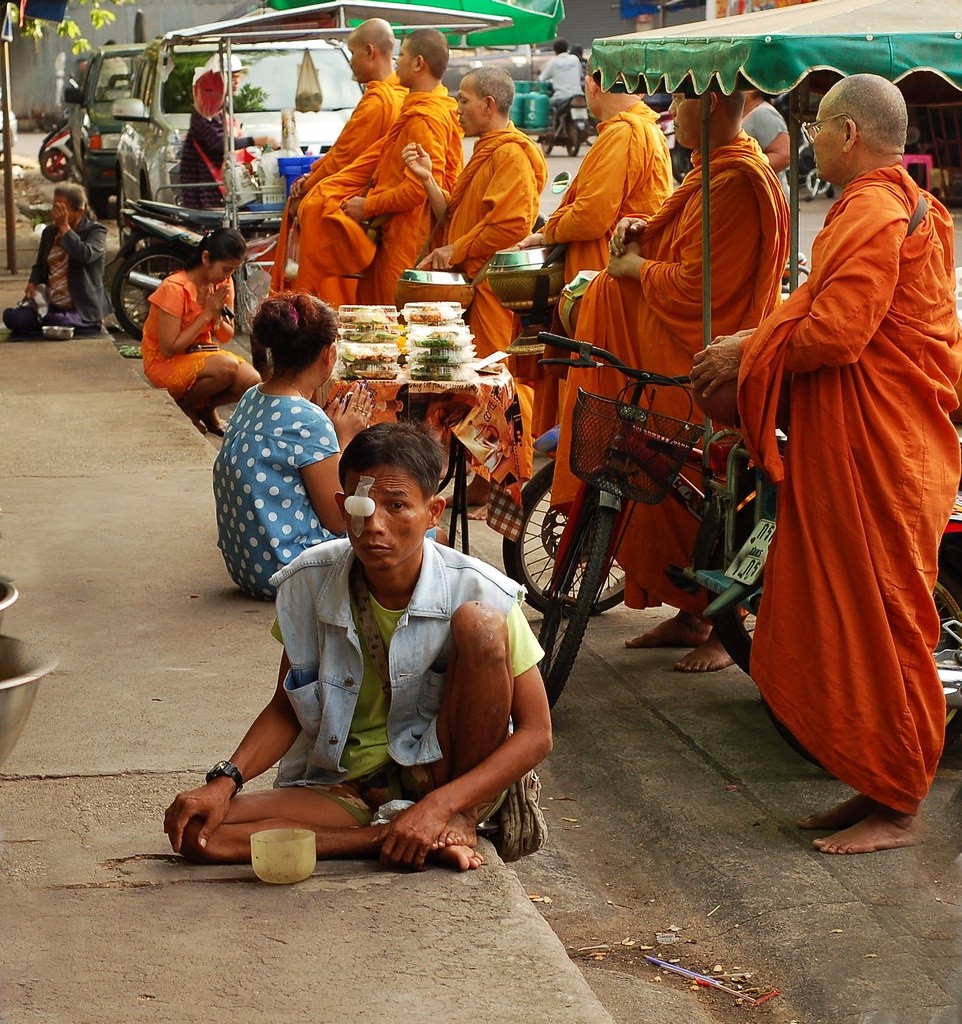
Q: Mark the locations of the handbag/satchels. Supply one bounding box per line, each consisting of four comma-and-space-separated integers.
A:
295, 45, 322, 113
499, 765, 548, 861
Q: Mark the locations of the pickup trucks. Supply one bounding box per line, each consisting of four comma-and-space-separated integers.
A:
391, 38, 593, 102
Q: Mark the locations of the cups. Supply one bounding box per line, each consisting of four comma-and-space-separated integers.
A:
281, 108, 298, 151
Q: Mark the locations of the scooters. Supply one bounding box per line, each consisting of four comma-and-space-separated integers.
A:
38, 78, 80, 182
641, 93, 693, 183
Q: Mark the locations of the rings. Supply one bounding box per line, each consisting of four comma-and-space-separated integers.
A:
362, 412, 367, 416
358, 407, 362, 411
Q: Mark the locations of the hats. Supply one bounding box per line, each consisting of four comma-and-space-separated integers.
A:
207, 52, 245, 74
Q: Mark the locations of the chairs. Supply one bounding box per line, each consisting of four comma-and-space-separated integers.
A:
107, 71, 132, 89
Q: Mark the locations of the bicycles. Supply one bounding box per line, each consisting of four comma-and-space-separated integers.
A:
502, 331, 961, 769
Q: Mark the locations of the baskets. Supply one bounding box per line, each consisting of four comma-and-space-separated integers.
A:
260, 186, 285, 206
570, 386, 709, 504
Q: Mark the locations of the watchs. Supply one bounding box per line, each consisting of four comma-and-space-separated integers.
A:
206, 761, 243, 797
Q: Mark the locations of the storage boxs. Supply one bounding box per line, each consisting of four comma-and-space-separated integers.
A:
243, 154, 319, 213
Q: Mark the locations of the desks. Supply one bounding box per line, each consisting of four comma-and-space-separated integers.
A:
322, 365, 515, 556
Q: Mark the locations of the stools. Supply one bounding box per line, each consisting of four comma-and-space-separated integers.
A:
904, 153, 935, 192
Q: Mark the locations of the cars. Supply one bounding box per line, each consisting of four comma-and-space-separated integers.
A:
63, 43, 151, 217
115, 38, 365, 250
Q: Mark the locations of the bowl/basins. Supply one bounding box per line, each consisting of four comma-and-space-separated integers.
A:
250, 829, 315, 884
42, 326, 75, 340
0, 575, 60, 774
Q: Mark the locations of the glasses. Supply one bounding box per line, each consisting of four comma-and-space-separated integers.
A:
802, 114, 858, 144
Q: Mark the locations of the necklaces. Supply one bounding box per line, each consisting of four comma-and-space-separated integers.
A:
274, 377, 304, 397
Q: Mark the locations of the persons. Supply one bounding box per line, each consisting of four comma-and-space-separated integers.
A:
569, 45, 588, 87
212, 291, 450, 602
163, 423, 554, 871
690, 73, 962, 855
3, 185, 107, 336
179, 52, 278, 240
538, 39, 585, 106
549, 90, 791, 672
742, 89, 791, 205
271, 18, 674, 520
141, 227, 262, 432
79, 40, 129, 87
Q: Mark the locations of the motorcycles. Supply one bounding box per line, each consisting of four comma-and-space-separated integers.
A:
104, 197, 282, 340
533, 80, 588, 156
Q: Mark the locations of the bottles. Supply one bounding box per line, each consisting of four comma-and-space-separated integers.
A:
224, 160, 257, 208
258, 144, 277, 187
